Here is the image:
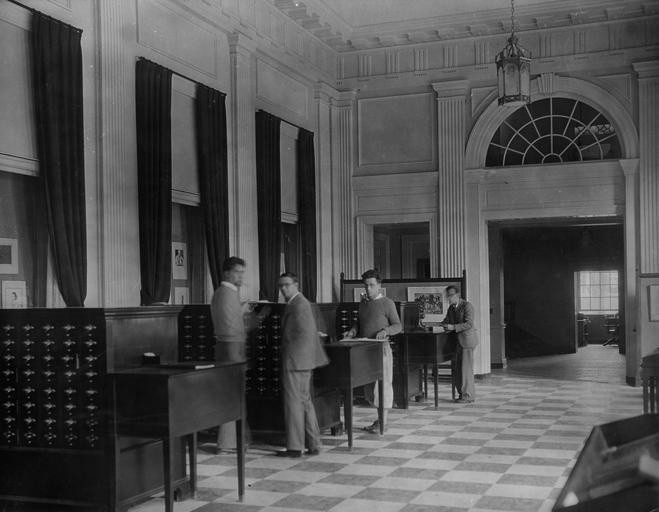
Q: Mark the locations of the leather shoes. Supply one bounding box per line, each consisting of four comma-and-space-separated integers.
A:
217, 449, 238, 454
455, 397, 474, 403
276, 451, 301, 457
364, 421, 387, 433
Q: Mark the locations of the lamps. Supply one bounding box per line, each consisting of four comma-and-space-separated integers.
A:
494, 1, 533, 109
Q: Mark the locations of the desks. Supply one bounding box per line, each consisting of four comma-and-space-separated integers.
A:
403, 328, 458, 408
313, 336, 385, 448
114, 359, 247, 512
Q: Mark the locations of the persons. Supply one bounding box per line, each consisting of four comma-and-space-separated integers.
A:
347, 268, 404, 434
276, 271, 332, 458
209, 256, 255, 455
438, 284, 479, 403
